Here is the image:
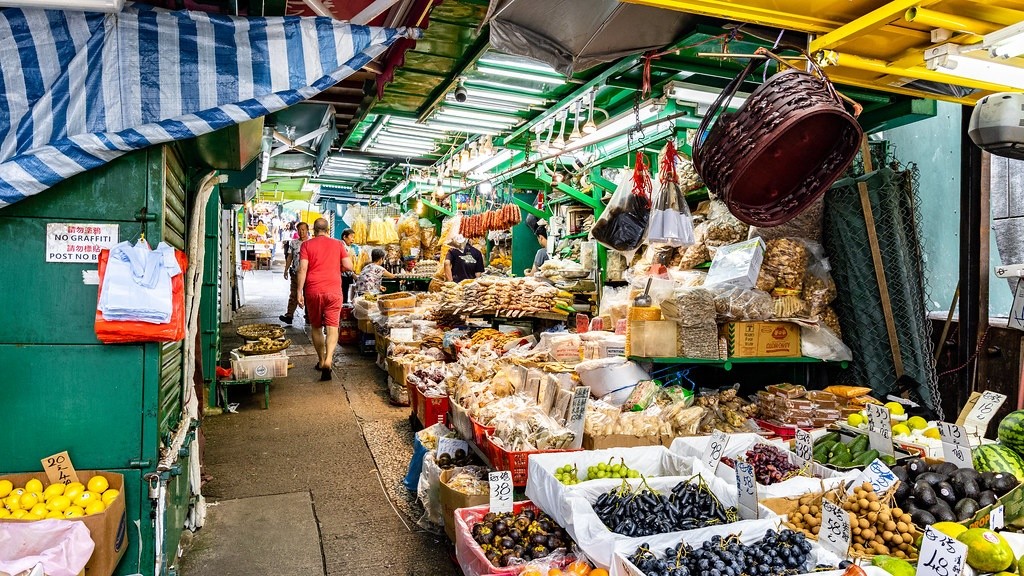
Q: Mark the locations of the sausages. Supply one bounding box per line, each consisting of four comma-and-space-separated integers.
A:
458, 203, 522, 240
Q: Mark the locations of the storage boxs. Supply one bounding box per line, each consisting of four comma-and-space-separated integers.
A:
0, 469, 129, 576
357, 318, 375, 333
254, 243, 274, 251
357, 329, 377, 359
660, 431, 705, 449
581, 428, 660, 451
385, 358, 395, 379
415, 384, 449, 430
356, 299, 381, 320
716, 321, 803, 357
229, 335, 290, 380
377, 291, 415, 311
392, 359, 447, 387
405, 378, 418, 415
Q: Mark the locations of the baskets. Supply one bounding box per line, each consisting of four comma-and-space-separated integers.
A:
468, 413, 496, 452
455, 499, 577, 576
449, 394, 472, 440
233, 355, 289, 379
487, 435, 585, 487
693, 44, 863, 227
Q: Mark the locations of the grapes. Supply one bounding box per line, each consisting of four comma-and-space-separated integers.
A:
627, 529, 854, 576
554, 456, 641, 485
720, 443, 822, 485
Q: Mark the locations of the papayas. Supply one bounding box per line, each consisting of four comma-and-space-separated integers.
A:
872, 521, 1024, 576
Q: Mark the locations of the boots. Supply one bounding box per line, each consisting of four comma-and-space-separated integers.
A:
314, 362, 323, 371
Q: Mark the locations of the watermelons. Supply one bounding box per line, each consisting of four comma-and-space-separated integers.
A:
971, 425, 1024, 482
998, 409, 1024, 455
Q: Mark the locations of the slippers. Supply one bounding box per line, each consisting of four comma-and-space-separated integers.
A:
321, 366, 332, 381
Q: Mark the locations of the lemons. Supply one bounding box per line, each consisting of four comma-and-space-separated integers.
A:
0, 476, 119, 520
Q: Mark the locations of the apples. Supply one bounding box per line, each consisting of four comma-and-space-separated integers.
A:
884, 402, 941, 440
847, 410, 867, 426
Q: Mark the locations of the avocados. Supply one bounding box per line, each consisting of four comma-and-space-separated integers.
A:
813, 433, 896, 466
891, 458, 1019, 526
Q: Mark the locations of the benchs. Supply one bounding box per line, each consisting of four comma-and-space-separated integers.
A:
219, 375, 272, 413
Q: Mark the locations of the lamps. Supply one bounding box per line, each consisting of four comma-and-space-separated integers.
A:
320, 87, 547, 177
388, 179, 410, 197
464, 171, 497, 181
408, 174, 469, 187
559, 96, 669, 152
552, 109, 569, 149
922, 42, 1024, 92
581, 92, 598, 133
470, 63, 569, 85
569, 101, 583, 141
537, 117, 556, 153
982, 20, 1024, 61
662, 80, 751, 110
478, 51, 561, 74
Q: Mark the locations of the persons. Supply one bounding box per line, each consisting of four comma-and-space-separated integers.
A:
283, 221, 298, 263
895, 374, 939, 421
338, 228, 357, 308
254, 219, 269, 236
524, 226, 554, 277
444, 238, 485, 283
279, 222, 312, 324
352, 249, 395, 303
297, 218, 353, 382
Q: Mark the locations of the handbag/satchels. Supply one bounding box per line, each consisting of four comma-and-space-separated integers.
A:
648, 171, 694, 245
592, 168, 654, 254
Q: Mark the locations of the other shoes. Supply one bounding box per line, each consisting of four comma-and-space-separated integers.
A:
305, 316, 310, 323
278, 315, 293, 324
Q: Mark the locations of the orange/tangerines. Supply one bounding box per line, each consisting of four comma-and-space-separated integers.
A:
523, 560, 609, 576
